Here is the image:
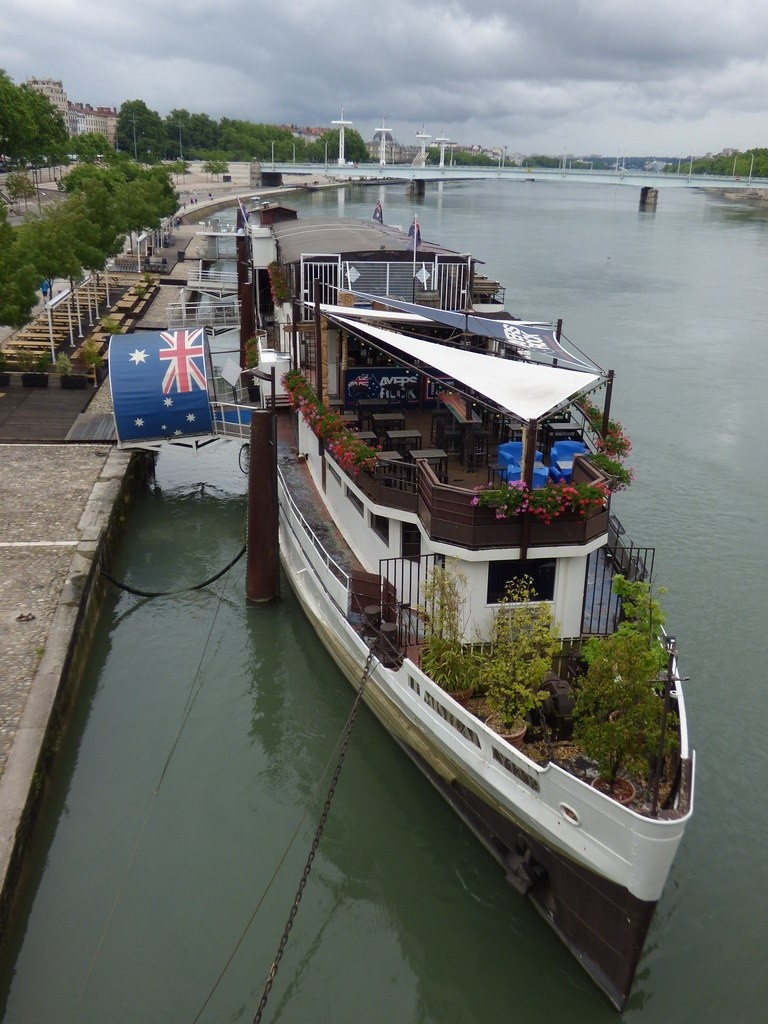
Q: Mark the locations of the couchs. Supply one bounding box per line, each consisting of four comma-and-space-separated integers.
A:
497, 441, 588, 488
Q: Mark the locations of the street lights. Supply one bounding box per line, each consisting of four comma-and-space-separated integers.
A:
271, 140, 277, 167
325, 141, 328, 163
291, 142, 295, 163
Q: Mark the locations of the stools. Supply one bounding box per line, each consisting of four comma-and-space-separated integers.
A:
432, 409, 489, 472
394, 440, 415, 450
508, 433, 522, 442
536, 441, 545, 463
487, 463, 507, 485
427, 459, 440, 464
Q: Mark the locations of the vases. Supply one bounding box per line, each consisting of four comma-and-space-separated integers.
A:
248, 385, 260, 403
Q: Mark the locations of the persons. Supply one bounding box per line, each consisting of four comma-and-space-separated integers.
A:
41, 279, 50, 305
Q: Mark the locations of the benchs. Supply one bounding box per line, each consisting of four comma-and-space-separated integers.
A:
4, 277, 159, 361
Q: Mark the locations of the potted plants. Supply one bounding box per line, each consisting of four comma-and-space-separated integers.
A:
0, 351, 13, 387
15, 349, 51, 387
412, 555, 681, 805
57, 347, 95, 389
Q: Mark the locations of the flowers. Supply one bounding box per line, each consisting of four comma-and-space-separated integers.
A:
570, 391, 637, 494
267, 260, 292, 307
470, 480, 611, 525
243, 335, 259, 371
280, 370, 383, 476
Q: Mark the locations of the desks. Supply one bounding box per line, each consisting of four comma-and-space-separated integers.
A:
108, 312, 127, 323
117, 301, 136, 312
328, 397, 449, 487
0, 272, 120, 362
129, 288, 144, 295
123, 295, 138, 302
134, 282, 148, 289
478, 395, 584, 464
140, 277, 152, 282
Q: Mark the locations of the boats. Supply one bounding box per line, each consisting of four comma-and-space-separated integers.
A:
256, 253, 697, 1010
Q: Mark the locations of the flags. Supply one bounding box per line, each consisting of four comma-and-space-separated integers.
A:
408, 216, 421, 247
373, 198, 383, 225
237, 196, 250, 225
